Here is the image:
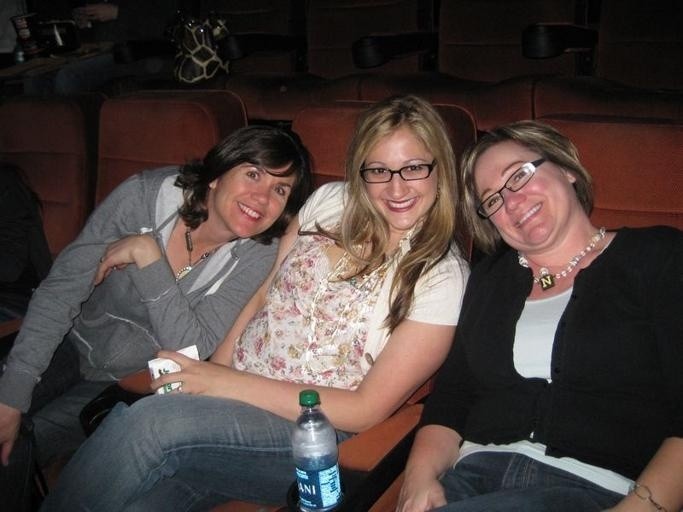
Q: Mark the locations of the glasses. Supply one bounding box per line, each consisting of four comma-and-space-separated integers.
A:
360, 157, 438, 183
476, 158, 547, 220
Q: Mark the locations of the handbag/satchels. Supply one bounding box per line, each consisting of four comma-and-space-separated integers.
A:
172, 9, 228, 90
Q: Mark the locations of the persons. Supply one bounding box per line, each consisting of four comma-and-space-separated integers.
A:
72, 0, 186, 64
1, 125, 316, 510
38, 96, 471, 510
393, 119, 683, 512
0, 0, 28, 94
0, 162, 52, 363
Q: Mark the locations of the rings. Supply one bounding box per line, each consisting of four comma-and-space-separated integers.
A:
177, 385, 183, 393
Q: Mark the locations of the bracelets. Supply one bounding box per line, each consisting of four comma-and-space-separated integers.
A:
629, 480, 669, 512
111, 4, 119, 20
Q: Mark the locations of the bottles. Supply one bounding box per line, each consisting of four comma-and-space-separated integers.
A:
290, 390, 342, 512
15, 37, 26, 65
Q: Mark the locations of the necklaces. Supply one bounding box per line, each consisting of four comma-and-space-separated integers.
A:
516, 227, 607, 290
305, 248, 403, 375
175, 226, 237, 283
